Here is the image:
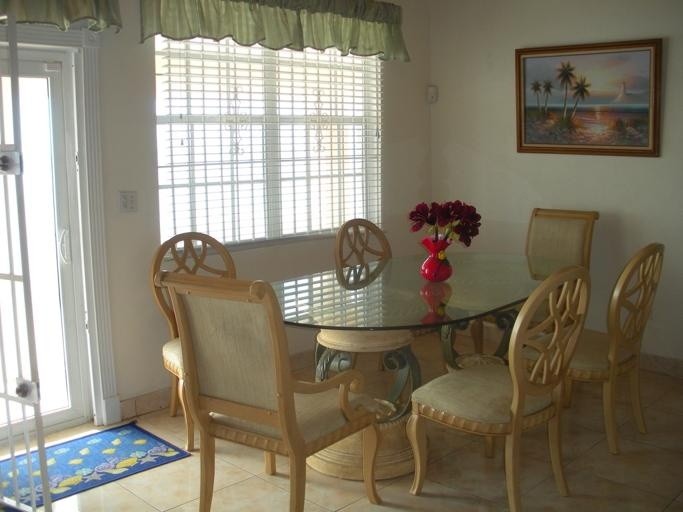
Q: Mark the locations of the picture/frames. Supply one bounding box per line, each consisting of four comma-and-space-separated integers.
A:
513, 37, 663, 160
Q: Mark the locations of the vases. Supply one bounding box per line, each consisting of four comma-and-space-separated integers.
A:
420, 236, 453, 282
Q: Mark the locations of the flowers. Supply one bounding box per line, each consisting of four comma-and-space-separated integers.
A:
409, 198, 482, 248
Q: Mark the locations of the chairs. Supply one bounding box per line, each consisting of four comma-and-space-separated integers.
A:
406, 265, 593, 511
336, 259, 388, 291
152, 268, 386, 512
515, 242, 665, 456
149, 231, 237, 418
335, 219, 392, 266
469, 210, 600, 354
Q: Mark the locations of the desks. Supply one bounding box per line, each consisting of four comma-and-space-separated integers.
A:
257, 258, 549, 486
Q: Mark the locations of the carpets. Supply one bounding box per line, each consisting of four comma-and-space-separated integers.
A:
1, 418, 189, 508
